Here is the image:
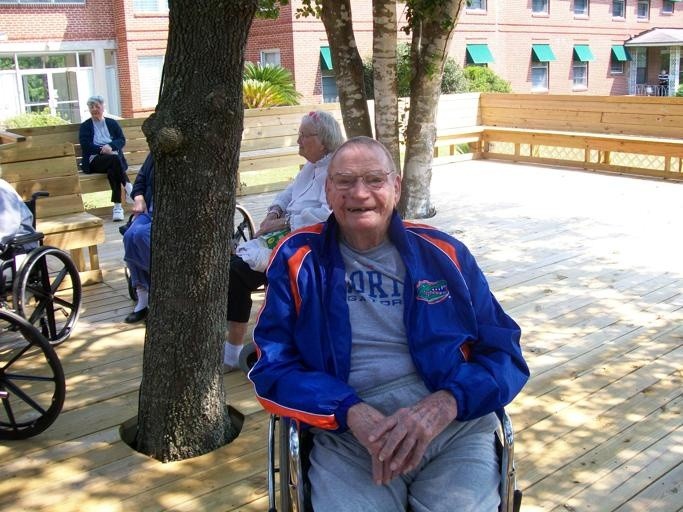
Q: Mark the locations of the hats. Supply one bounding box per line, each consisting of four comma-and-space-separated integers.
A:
87, 96, 103, 105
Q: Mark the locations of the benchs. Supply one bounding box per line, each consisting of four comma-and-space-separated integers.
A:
477, 91, 682, 182
0, 143, 107, 293
1, 92, 479, 219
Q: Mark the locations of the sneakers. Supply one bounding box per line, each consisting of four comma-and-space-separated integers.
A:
125, 307, 148, 323
112, 207, 124, 221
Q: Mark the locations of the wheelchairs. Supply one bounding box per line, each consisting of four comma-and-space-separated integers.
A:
0, 192, 81, 346
118, 202, 259, 302
1, 311, 68, 442
266, 401, 523, 507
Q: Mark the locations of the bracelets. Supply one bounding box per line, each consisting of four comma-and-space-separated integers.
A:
284, 215, 290, 229
267, 208, 280, 219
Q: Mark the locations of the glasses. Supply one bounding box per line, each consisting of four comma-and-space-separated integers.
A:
298, 133, 319, 139
328, 171, 394, 188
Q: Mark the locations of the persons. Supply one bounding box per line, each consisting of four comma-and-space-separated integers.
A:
0, 174, 43, 286
656, 68, 668, 96
78, 95, 139, 222
217, 108, 349, 377
118, 150, 163, 325
247, 136, 531, 510
